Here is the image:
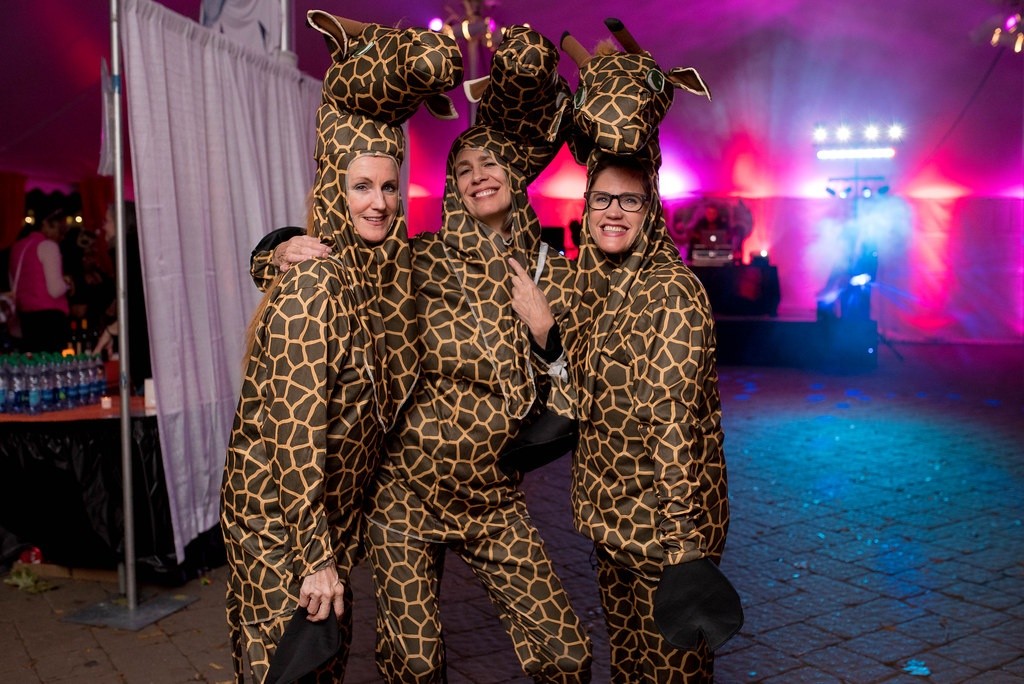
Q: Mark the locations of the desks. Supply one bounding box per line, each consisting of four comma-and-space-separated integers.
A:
0, 397, 160, 600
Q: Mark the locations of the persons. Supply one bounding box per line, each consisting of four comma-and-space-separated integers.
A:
7, 186, 148, 395
555, 151, 744, 684
249, 127, 595, 684
221, 124, 463, 684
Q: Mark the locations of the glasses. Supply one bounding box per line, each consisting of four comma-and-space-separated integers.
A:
583, 191, 649, 212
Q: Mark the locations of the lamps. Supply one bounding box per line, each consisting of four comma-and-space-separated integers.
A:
812, 143, 900, 160
840, 187, 851, 198
846, 266, 871, 287
863, 187, 871, 199
876, 186, 889, 194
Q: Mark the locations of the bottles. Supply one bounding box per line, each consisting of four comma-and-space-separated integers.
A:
0, 352, 105, 415
